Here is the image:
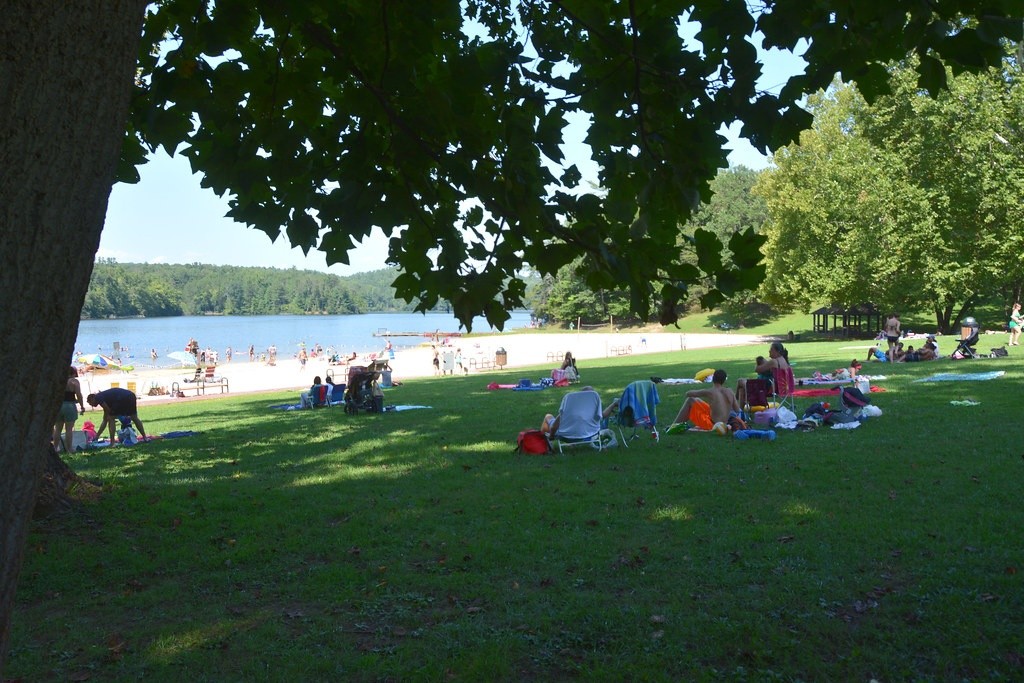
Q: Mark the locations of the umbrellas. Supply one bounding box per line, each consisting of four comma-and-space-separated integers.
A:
78, 354, 117, 381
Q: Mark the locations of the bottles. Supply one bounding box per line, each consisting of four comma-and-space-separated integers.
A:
680, 422, 688, 433
990, 353, 994, 359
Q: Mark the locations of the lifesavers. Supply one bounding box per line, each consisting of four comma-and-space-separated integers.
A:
744, 401, 780, 412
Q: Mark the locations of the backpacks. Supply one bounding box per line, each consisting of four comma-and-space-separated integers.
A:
844, 388, 871, 407
515, 430, 553, 454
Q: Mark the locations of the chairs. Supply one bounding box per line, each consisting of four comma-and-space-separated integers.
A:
745, 366, 796, 418
609, 380, 661, 448
551, 391, 602, 455
326, 383, 346, 404
305, 384, 331, 410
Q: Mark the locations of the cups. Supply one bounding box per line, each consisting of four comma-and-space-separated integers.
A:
799, 379, 803, 386
652, 432, 659, 439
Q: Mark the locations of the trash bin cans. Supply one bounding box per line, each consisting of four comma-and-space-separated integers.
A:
962, 318, 980, 339
495, 347, 507, 366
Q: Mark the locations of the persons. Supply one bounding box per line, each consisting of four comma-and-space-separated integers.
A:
324, 377, 335, 396
431, 345, 440, 373
53, 366, 85, 452
117, 416, 138, 446
301, 375, 323, 408
86, 387, 150, 445
867, 313, 937, 363
455, 348, 462, 370
736, 341, 794, 410
374, 363, 392, 389
186, 337, 205, 361
385, 340, 392, 351
297, 343, 356, 370
225, 344, 276, 362
541, 386, 621, 434
151, 349, 157, 359
667, 369, 740, 429
806, 358, 861, 379
561, 352, 578, 374
1008, 303, 1024, 346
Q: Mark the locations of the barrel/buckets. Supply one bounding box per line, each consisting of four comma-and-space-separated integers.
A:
111, 381, 136, 392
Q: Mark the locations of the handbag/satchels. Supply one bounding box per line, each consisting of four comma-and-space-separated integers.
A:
117, 428, 138, 446
59, 426, 88, 453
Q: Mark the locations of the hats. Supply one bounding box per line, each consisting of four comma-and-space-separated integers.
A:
926, 335, 935, 339
72, 366, 78, 378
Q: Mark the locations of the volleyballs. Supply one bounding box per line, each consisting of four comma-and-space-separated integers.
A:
713, 422, 728, 436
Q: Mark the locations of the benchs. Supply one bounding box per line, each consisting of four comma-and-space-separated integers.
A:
171, 377, 228, 397
547, 351, 564, 362
326, 359, 389, 381
469, 356, 491, 368
611, 345, 633, 356
